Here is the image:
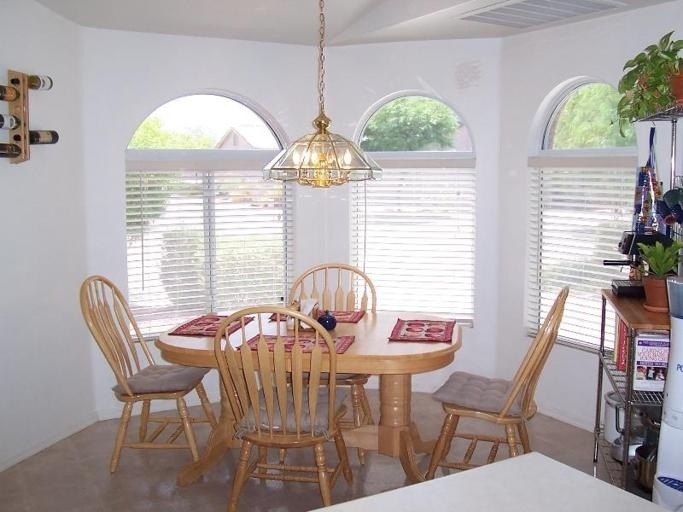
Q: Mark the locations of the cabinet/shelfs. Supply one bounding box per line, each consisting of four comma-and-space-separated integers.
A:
592, 288, 676, 500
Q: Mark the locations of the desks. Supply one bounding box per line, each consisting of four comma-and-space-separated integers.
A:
300, 449, 673, 512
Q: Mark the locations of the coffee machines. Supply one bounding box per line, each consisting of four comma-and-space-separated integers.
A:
603, 229, 675, 296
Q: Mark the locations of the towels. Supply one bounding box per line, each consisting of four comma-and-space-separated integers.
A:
388, 316, 457, 346
236, 332, 356, 355
266, 309, 365, 326
168, 313, 252, 340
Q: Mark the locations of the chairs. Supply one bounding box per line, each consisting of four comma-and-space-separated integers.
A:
207, 302, 357, 512
75, 272, 223, 474
285, 259, 378, 466
418, 278, 570, 483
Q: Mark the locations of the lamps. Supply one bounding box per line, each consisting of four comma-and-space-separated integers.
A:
259, 1, 386, 188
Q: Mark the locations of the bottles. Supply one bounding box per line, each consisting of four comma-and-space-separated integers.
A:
317, 309, 336, 329
286, 314, 296, 331
12, 128, 59, 144
0, 113, 21, 129
12, 72, 54, 92
0, 143, 22, 157
0, 84, 19, 102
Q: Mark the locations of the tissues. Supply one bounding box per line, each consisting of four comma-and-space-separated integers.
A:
298, 298, 319, 332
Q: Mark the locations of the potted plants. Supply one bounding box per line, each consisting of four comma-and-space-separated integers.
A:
609, 26, 682, 136
637, 238, 682, 316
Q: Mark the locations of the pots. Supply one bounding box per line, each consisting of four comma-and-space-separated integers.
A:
629, 443, 654, 491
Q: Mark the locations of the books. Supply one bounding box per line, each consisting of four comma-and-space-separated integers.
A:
610, 312, 670, 392
